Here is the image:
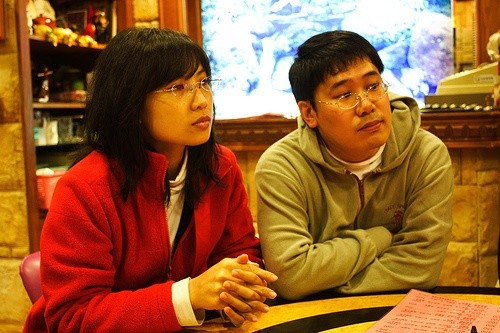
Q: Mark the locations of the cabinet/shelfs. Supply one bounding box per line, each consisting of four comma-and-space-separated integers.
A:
15, 0, 134, 256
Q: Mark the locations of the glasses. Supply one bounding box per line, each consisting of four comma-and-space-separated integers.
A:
151, 73, 221, 98
314, 79, 391, 110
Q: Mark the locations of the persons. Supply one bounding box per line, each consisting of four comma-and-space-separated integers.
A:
20, 26, 280, 333
254, 30, 453, 299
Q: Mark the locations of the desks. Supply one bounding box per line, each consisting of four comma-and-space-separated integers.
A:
182, 286, 500, 333
